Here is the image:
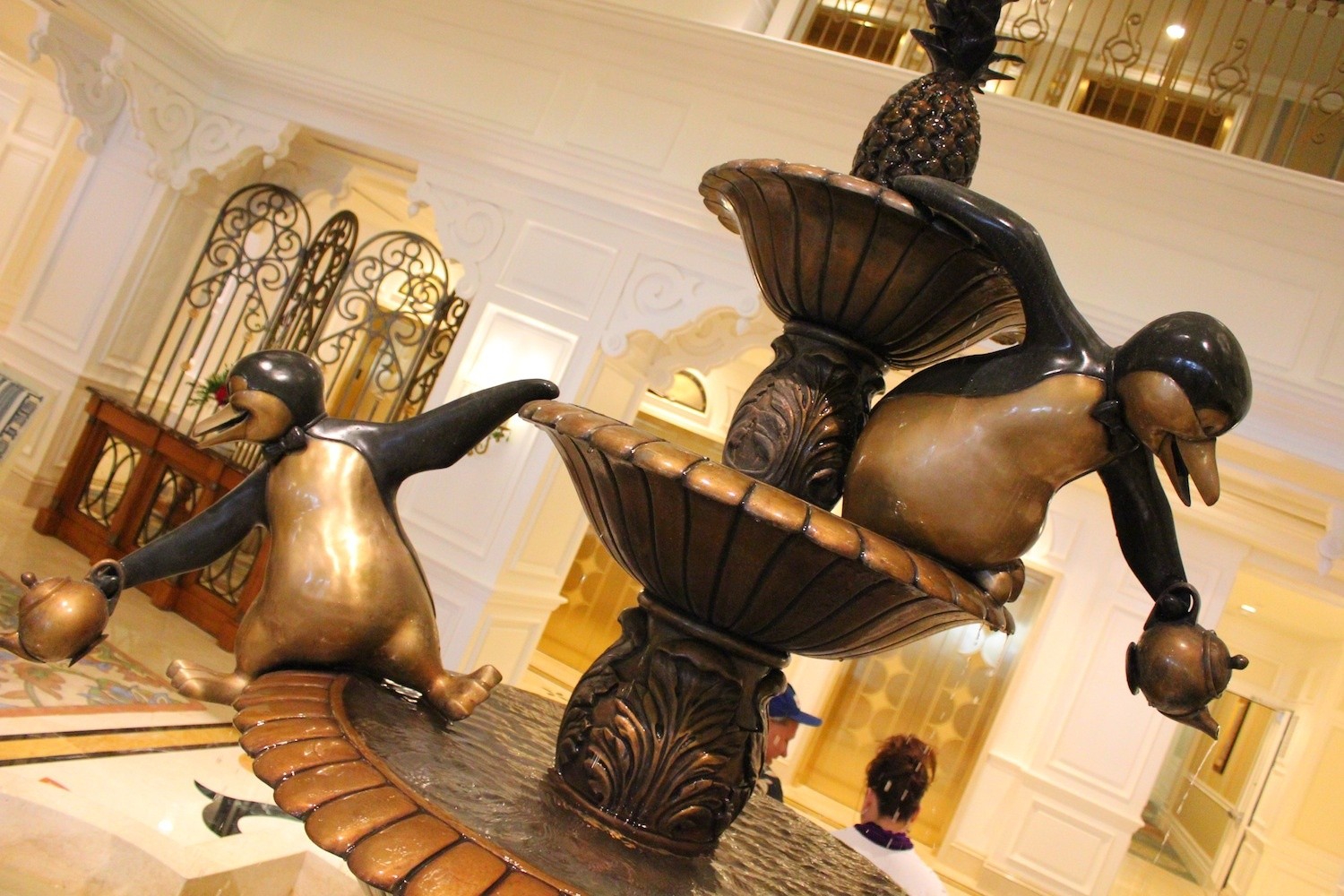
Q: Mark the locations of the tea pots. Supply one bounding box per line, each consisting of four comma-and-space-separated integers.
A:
1, 558, 128, 669
1125, 580, 1247, 742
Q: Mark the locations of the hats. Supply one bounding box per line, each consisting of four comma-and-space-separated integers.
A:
766, 682, 822, 726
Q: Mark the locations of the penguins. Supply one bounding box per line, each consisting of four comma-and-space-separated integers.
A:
92, 350, 563, 724
841, 176, 1254, 631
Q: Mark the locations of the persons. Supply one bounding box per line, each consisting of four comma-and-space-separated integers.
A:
832, 733, 946, 896
757, 682, 822, 802
